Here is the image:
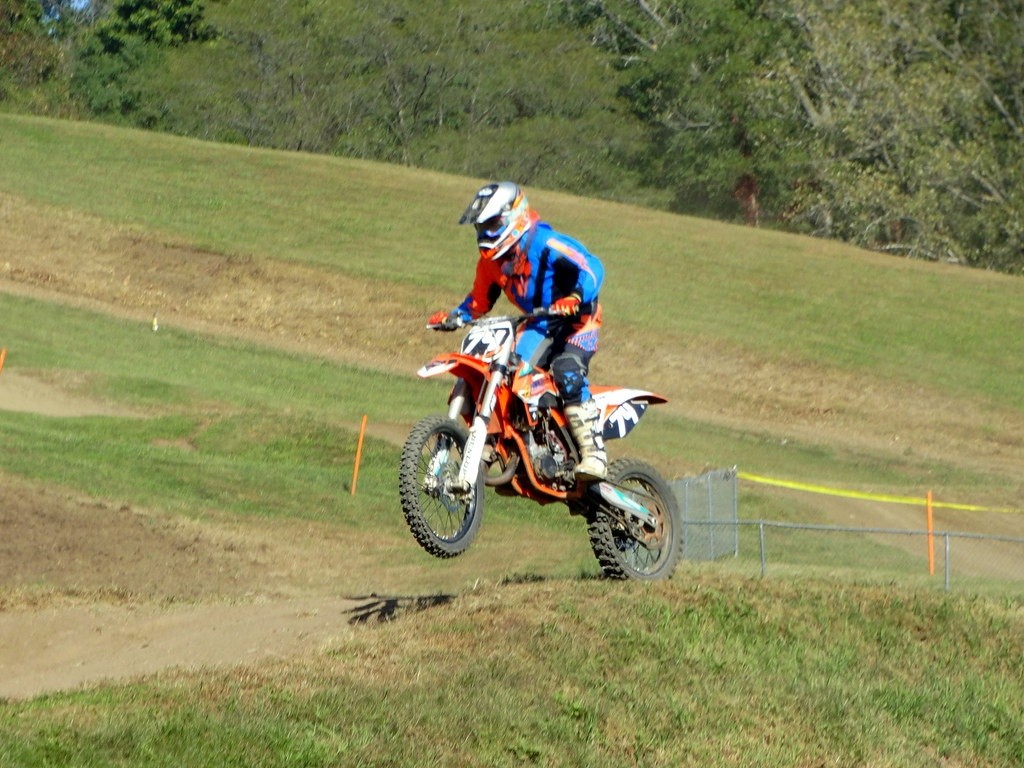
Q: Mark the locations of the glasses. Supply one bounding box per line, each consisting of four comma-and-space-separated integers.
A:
474, 212, 513, 241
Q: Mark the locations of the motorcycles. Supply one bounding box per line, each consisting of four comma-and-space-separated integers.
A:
396, 300, 686, 580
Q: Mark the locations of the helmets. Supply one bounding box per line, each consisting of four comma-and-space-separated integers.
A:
458, 180, 540, 260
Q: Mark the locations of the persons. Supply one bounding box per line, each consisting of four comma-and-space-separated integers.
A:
431, 182, 607, 494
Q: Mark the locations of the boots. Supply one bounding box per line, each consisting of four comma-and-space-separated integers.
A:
564, 398, 607, 481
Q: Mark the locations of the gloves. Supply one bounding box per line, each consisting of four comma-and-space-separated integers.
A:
429, 311, 465, 330
548, 292, 582, 315
